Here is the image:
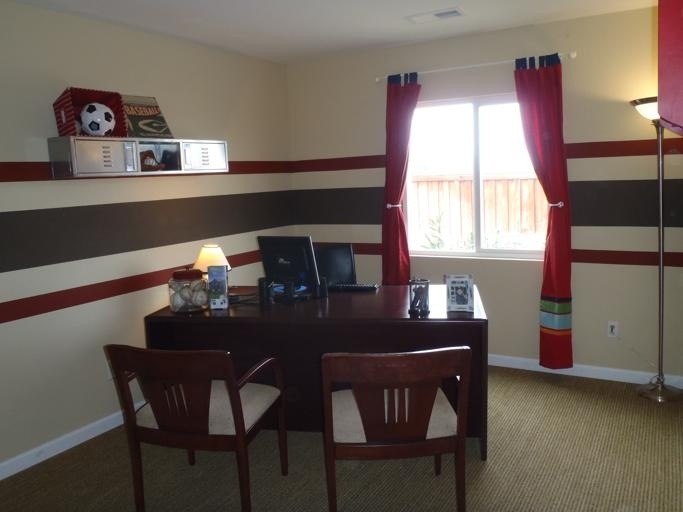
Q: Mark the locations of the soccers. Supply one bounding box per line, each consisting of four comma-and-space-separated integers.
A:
78, 102, 116, 136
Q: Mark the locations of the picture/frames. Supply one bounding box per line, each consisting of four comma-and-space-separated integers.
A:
447, 274, 474, 312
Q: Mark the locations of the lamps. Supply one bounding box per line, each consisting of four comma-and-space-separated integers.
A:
193, 244, 232, 283
630, 94, 682, 404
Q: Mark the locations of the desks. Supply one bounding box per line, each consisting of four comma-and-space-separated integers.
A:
143, 288, 488, 462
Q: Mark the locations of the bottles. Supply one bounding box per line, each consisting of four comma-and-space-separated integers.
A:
166, 266, 210, 314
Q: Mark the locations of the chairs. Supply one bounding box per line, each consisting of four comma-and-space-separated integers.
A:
311, 241, 356, 285
321, 346, 473, 511
104, 342, 288, 511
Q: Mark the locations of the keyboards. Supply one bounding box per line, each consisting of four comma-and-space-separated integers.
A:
327, 281, 380, 291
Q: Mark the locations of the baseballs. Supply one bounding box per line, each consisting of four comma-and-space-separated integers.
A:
168, 279, 207, 309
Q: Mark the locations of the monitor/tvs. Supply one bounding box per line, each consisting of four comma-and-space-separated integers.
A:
256, 235, 321, 303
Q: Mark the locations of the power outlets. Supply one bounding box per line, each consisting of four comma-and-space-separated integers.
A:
608, 320, 617, 337
